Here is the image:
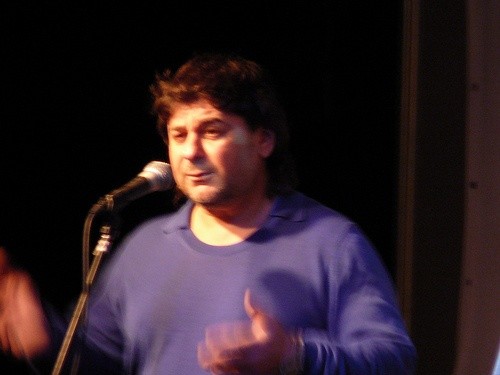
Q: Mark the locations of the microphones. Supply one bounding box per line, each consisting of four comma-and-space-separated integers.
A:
101, 160, 175, 208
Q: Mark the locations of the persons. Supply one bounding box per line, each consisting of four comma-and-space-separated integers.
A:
74, 56, 419, 375
0, 247, 52, 375
